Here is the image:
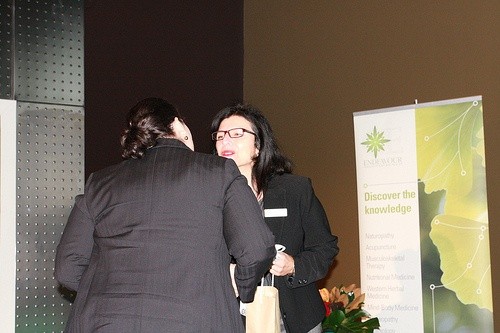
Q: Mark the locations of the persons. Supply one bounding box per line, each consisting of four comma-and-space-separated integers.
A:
208, 102, 339, 333
54, 96, 278, 333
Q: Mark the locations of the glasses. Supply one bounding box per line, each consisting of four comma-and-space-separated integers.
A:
211, 128, 256, 141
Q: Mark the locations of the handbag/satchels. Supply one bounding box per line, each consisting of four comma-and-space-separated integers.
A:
241, 272, 281, 333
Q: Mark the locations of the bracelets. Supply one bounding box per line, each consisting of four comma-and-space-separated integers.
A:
286, 268, 296, 287
